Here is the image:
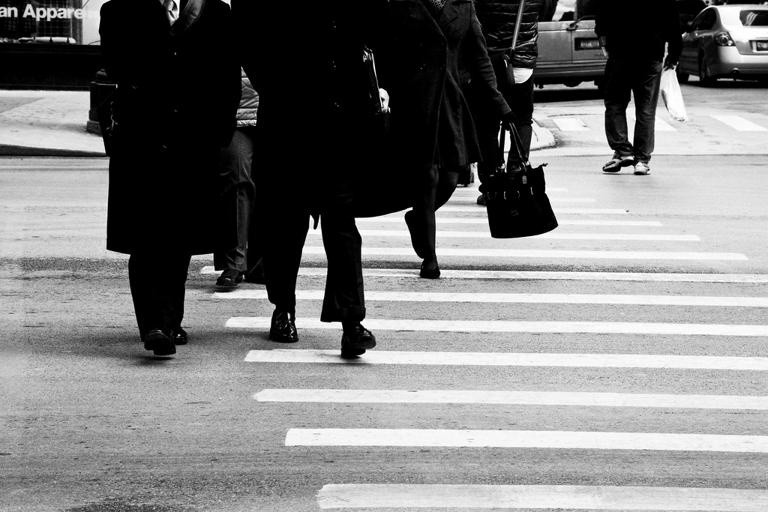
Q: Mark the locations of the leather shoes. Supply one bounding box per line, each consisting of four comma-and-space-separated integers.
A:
142, 327, 187, 356
340, 323, 376, 360
216, 269, 241, 287
269, 309, 299, 343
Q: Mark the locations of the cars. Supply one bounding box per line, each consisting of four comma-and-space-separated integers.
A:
674, 1, 767, 89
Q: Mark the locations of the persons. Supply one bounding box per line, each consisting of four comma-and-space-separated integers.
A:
587, 1, 684, 173
93, 0, 538, 364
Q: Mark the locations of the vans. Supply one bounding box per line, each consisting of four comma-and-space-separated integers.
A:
532, 0, 610, 93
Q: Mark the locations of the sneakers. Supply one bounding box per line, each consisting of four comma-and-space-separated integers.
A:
419, 261, 441, 280
602, 152, 650, 175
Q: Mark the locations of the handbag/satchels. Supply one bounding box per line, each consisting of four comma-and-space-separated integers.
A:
490, 46, 516, 93
480, 118, 559, 238
